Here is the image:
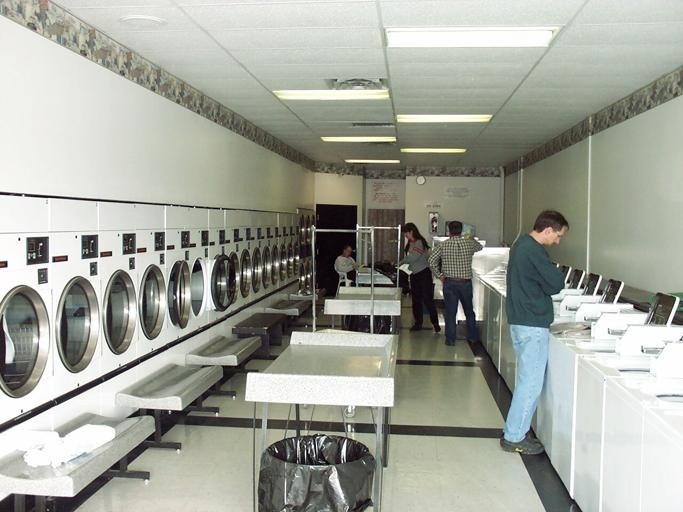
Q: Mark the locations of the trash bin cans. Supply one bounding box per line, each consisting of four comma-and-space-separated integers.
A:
258, 434, 377, 512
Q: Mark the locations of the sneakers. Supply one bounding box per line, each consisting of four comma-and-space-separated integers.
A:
503, 438, 544, 455
500, 431, 541, 446
408, 323, 423, 331
435, 324, 442, 332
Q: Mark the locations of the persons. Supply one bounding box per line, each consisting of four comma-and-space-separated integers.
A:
500, 209, 569, 455
335, 245, 359, 282
428, 221, 483, 346
400, 222, 441, 333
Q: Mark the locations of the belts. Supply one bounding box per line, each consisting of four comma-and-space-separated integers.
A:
443, 277, 473, 283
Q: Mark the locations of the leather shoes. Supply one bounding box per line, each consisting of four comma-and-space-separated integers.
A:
445, 338, 457, 346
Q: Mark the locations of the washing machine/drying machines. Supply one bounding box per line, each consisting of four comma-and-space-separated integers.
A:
310, 214, 316, 257
135, 228, 169, 363
165, 227, 191, 344
271, 226, 281, 294
288, 225, 296, 284
534, 293, 680, 501
227, 227, 243, 317
252, 227, 265, 304
298, 208, 306, 257
51, 229, 104, 404
279, 226, 288, 287
0, 233, 53, 432
305, 259, 312, 288
488, 264, 602, 375
240, 226, 255, 310
603, 330, 683, 512
499, 278, 625, 444
261, 227, 272, 299
299, 263, 306, 291
207, 229, 236, 326
304, 210, 311, 256
295, 224, 303, 281
480, 263, 587, 375
187, 228, 210, 336
97, 226, 139, 377
575, 314, 683, 511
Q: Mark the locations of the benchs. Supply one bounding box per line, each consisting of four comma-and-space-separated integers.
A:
0, 411, 157, 512
187, 334, 260, 397
116, 363, 224, 451
265, 298, 311, 326
232, 314, 288, 358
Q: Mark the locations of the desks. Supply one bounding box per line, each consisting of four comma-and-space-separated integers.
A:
244, 331, 399, 512
324, 287, 402, 333
